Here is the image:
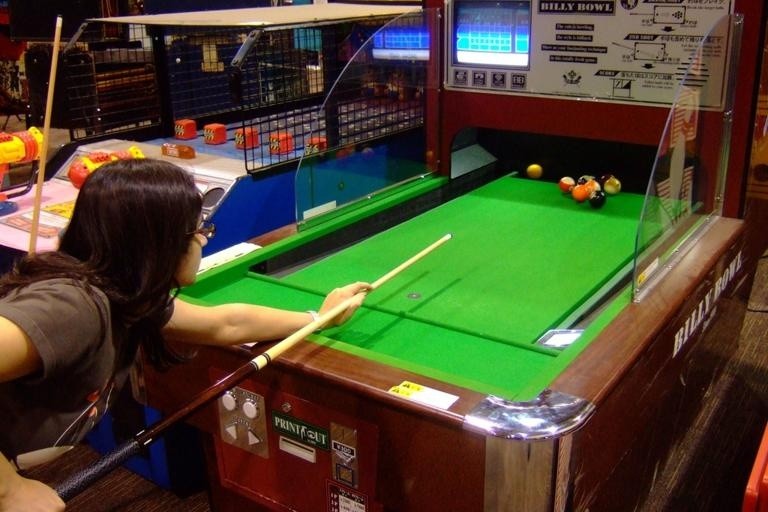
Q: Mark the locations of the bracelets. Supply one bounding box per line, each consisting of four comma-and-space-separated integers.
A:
307, 309, 322, 332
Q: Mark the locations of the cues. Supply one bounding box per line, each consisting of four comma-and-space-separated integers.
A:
52, 233, 452, 495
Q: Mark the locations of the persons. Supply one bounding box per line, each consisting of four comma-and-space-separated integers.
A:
0, 160, 374, 512
8, 60, 19, 99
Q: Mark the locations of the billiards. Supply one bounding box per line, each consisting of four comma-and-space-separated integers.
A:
526, 163, 544, 176
559, 173, 622, 208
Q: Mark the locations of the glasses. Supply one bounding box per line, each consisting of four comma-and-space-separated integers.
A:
185, 220, 216, 239
